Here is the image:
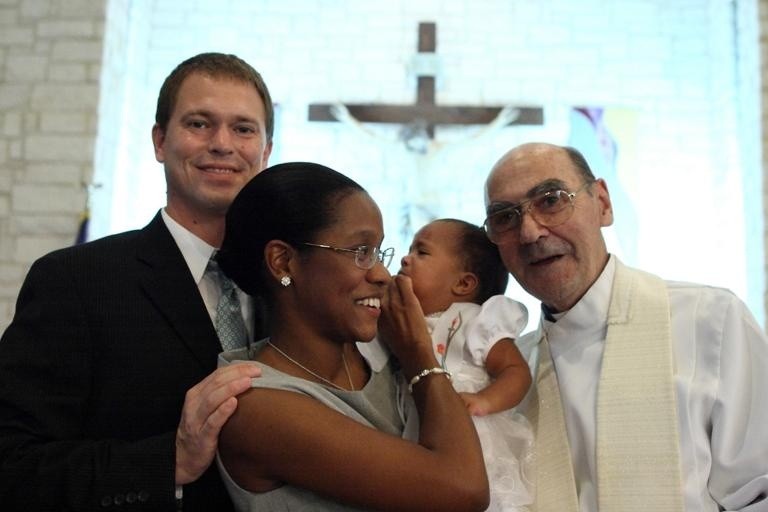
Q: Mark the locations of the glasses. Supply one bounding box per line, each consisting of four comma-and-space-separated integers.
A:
482, 188, 584, 247
302, 242, 394, 270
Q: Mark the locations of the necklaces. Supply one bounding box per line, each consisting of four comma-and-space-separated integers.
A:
265, 335, 355, 391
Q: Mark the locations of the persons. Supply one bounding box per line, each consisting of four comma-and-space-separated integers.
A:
209, 161, 491, 512
477, 141, 768, 512
391, 218, 537, 512
0, 52, 276, 511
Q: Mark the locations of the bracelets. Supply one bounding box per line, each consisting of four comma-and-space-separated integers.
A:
407, 363, 454, 392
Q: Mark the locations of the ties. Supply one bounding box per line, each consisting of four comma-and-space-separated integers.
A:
204, 248, 250, 353
